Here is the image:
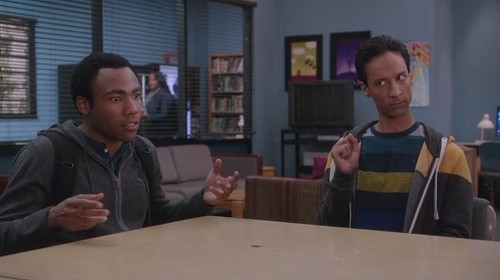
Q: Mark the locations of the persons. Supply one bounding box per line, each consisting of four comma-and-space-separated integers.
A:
315, 35, 473, 241
0, 51, 239, 254
140, 68, 175, 134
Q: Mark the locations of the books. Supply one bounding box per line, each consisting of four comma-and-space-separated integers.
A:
212, 74, 243, 91
214, 115, 241, 132
212, 57, 244, 73
212, 94, 243, 112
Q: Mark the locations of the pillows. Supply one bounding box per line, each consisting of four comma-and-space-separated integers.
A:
307, 157, 326, 178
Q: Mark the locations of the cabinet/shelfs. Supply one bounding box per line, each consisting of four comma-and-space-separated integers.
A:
208, 53, 243, 139
281, 127, 351, 179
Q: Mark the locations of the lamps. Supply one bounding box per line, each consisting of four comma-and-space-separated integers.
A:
475, 114, 493, 143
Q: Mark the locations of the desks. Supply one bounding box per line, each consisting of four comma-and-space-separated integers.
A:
0, 214, 500, 280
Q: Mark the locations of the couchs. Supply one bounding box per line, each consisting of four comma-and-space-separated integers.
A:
156, 143, 262, 213
242, 176, 327, 225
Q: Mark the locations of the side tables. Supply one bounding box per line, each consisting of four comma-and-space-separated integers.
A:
262, 166, 275, 176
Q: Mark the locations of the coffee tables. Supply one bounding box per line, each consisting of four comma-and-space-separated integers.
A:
226, 188, 245, 218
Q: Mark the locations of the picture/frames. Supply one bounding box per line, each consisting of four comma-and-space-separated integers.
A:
329, 30, 372, 90
284, 33, 323, 91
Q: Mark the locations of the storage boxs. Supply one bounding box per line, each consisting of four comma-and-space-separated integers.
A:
302, 151, 328, 165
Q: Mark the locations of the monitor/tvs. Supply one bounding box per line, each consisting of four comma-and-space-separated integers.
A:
288, 80, 354, 132
